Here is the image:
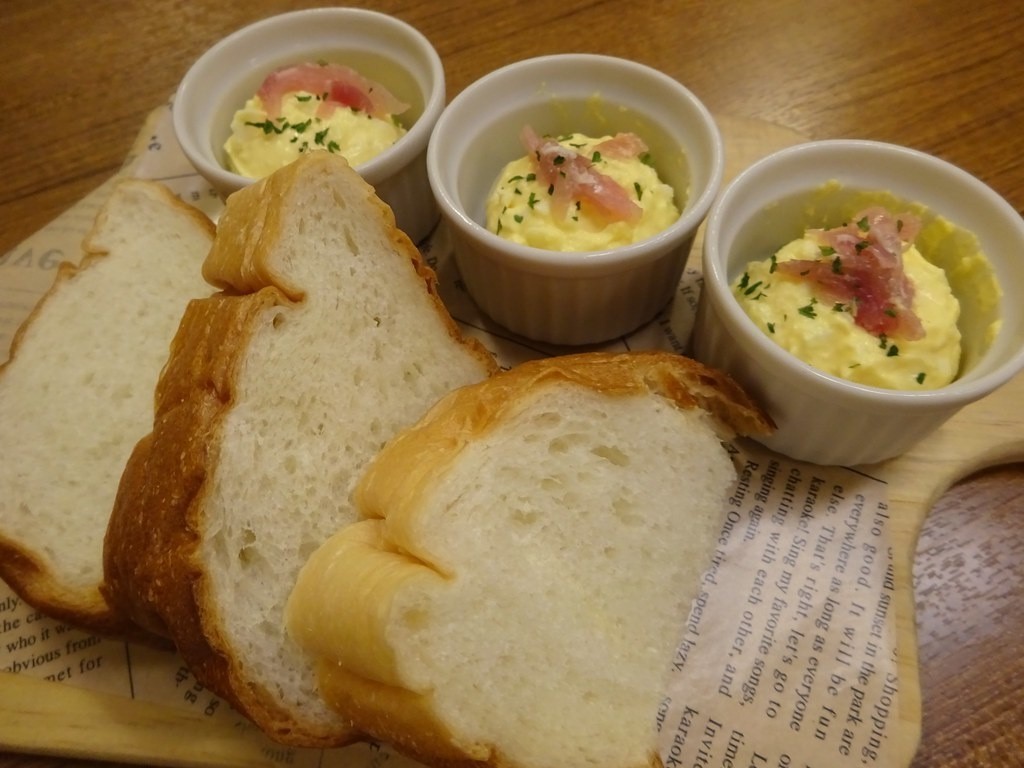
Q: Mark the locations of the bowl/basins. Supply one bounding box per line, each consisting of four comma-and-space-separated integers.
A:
690, 142, 1024, 466
426, 53, 722, 343
172, 9, 445, 248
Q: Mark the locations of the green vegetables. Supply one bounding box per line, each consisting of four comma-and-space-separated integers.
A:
496, 131, 644, 240
735, 214, 926, 386
241, 92, 374, 155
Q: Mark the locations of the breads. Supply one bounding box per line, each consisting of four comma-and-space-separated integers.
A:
0, 176, 220, 650
97, 148, 506, 749
280, 348, 782, 768
